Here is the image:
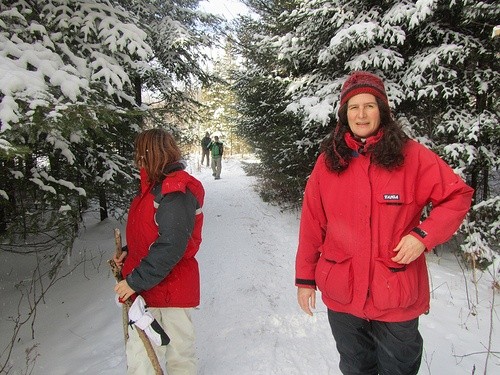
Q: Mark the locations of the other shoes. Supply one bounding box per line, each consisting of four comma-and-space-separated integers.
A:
214, 177, 220, 180
201, 161, 203, 164
212, 173, 216, 176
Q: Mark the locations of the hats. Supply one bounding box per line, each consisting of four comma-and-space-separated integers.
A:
338, 71, 391, 116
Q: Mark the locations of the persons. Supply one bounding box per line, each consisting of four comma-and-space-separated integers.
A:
113, 129, 205, 375
200, 131, 212, 168
209, 136, 224, 180
294, 70, 474, 375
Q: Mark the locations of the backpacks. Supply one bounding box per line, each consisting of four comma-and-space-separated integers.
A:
211, 142, 222, 156
